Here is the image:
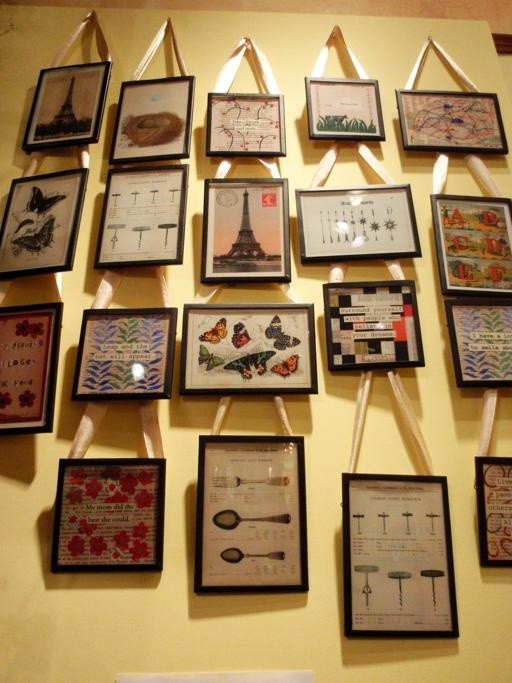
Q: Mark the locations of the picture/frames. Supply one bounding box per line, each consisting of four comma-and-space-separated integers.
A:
21, 61, 113, 151
180, 304, 318, 395
2, 301, 64, 435
342, 472, 459, 639
295, 184, 422, 266
205, 93, 287, 157
305, 76, 386, 142
92, 165, 189, 269
71, 306, 178, 401
200, 178, 291, 284
430, 194, 511, 389
323, 280, 425, 372
2, 167, 90, 279
50, 456, 166, 574
474, 457, 511, 568
193, 435, 309, 596
396, 88, 509, 154
108, 75, 196, 164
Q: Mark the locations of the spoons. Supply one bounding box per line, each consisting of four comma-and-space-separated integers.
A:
218, 548, 289, 561
213, 505, 294, 530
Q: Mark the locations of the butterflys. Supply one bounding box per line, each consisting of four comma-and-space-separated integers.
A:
224, 351, 277, 380
232, 322, 252, 348
198, 317, 228, 344
199, 344, 226, 370
29, 186, 66, 215
265, 315, 301, 350
271, 354, 299, 376
12, 217, 55, 252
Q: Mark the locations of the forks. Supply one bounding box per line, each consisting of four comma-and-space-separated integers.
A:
213, 475, 292, 489
